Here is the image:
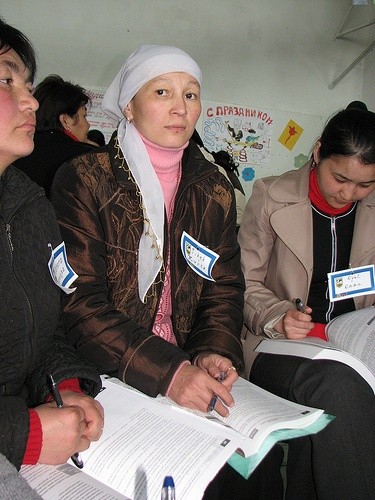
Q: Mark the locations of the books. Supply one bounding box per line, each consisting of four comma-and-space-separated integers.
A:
254, 305, 375, 396
19, 374, 245, 500
157, 376, 324, 458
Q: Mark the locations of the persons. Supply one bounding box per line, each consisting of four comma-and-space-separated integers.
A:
237, 102, 375, 500
0, 18, 285, 500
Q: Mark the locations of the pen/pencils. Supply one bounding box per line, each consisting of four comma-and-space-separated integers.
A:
160, 476, 175, 500
296, 298, 306, 313
48, 373, 84, 469
208, 371, 225, 411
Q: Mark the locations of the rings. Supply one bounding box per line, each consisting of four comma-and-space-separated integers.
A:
226, 366, 236, 373
